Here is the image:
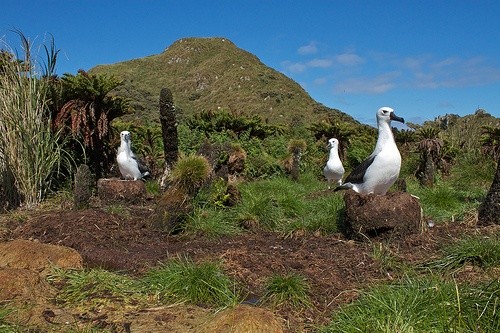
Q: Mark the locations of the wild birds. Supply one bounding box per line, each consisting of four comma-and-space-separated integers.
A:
329, 107, 408, 201
115, 131, 150, 182
323, 138, 346, 191
